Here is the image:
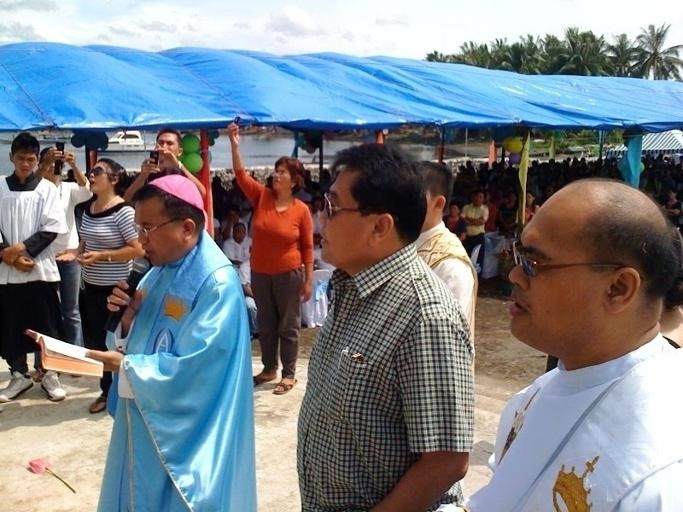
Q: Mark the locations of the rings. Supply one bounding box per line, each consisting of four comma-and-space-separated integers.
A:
107, 297, 110, 303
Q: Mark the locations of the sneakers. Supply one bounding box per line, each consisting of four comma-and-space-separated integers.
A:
40, 369, 67, 402
0, 371, 34, 403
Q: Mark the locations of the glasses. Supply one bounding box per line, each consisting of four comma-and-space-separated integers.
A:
512, 239, 623, 278
88, 166, 114, 178
131, 217, 189, 239
322, 190, 401, 223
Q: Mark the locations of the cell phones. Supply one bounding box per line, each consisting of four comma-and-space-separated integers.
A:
234, 116, 241, 126
55, 141, 65, 154
149, 150, 160, 166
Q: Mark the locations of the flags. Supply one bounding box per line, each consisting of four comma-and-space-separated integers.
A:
513, 130, 531, 227
485, 140, 499, 168
613, 132, 644, 191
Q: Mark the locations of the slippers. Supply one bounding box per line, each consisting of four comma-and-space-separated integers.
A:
88, 397, 107, 413
273, 377, 297, 395
253, 370, 277, 387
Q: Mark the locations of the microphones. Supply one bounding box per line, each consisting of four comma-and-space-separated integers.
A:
104, 256, 150, 333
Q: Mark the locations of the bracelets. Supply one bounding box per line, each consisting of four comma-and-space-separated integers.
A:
102, 248, 112, 265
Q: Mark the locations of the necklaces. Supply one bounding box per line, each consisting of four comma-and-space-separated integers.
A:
92, 195, 119, 213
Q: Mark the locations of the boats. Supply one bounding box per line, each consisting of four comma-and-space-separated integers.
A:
95, 130, 156, 153
11, 129, 74, 142
215, 126, 265, 135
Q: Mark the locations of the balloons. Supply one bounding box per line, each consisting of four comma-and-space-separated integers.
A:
291, 128, 336, 154
174, 129, 221, 174
489, 126, 531, 166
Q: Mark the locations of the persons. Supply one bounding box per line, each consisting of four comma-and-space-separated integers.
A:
51, 155, 147, 418
31, 144, 94, 383
431, 173, 683, 512
444, 160, 567, 274
564, 154, 681, 242
412, 158, 478, 356
0, 131, 71, 406
80, 172, 260, 512
292, 169, 333, 269
64, 169, 141, 195
292, 141, 477, 511
120, 129, 206, 209
225, 121, 317, 397
211, 175, 259, 337
